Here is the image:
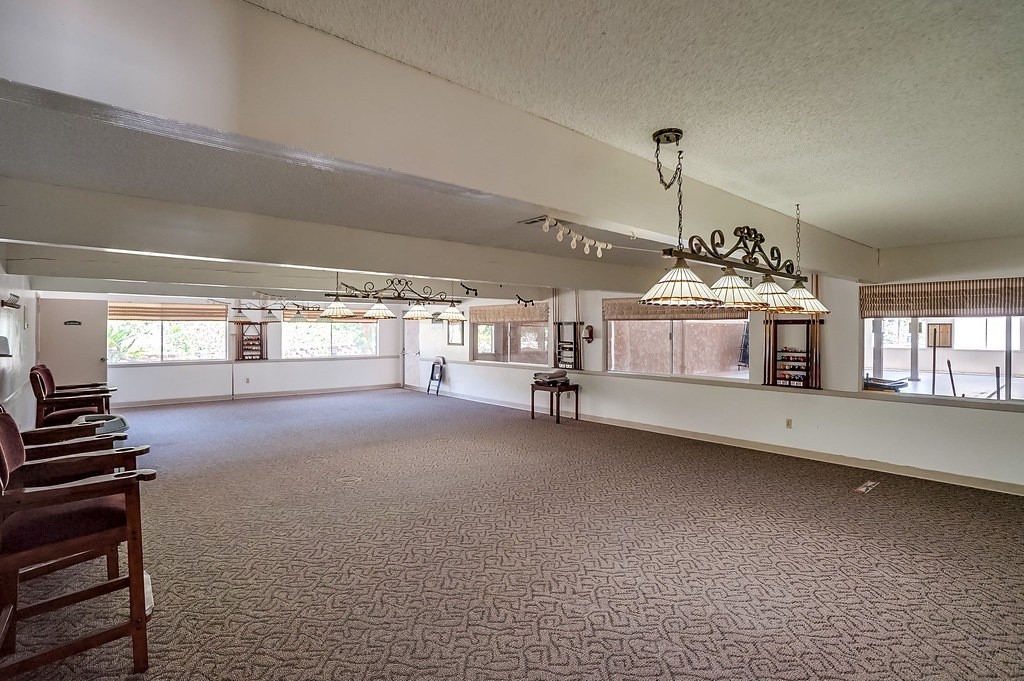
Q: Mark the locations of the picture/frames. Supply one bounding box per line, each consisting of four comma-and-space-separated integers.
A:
431, 314, 443, 323
447, 311, 465, 346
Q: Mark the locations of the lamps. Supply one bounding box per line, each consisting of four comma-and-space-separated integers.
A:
582, 324, 594, 344
228, 290, 335, 325
319, 270, 467, 323
635, 128, 831, 316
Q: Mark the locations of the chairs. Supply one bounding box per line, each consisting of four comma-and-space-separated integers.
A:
426, 356, 446, 396
0, 364, 157, 681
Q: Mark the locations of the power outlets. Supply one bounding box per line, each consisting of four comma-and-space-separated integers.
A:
566, 392, 570, 399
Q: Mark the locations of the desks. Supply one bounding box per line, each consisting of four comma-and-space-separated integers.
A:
529, 383, 580, 425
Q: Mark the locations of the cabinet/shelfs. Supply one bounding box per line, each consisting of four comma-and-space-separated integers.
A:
234, 324, 269, 361
762, 318, 824, 390
553, 321, 584, 371
